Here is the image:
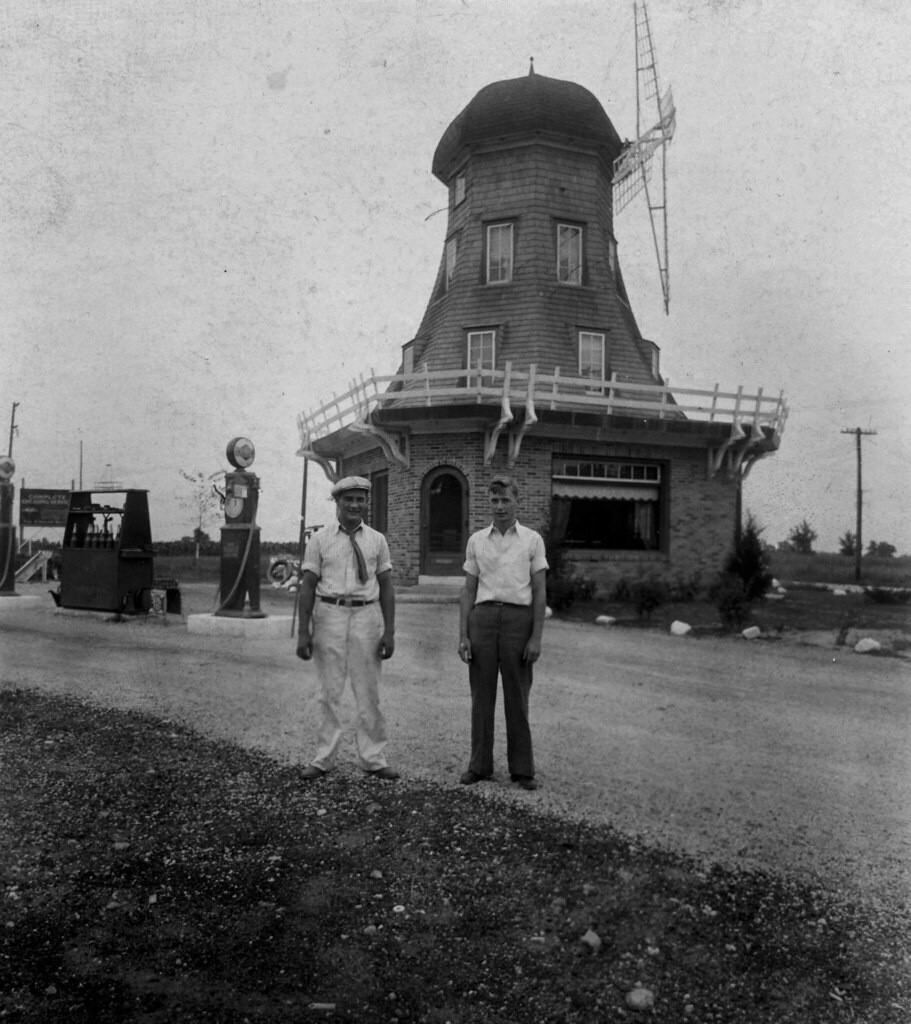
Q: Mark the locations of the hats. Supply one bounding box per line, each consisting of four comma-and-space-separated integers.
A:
332, 476, 372, 496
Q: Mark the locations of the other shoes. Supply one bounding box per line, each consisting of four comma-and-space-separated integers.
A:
299, 764, 327, 780
461, 766, 485, 784
366, 764, 400, 779
515, 773, 538, 791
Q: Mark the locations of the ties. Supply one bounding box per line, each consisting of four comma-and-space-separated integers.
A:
339, 526, 369, 587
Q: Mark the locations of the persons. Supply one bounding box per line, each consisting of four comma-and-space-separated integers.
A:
296, 476, 398, 780
458, 475, 550, 790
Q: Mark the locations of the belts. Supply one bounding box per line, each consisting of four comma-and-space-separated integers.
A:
320, 595, 374, 608
481, 600, 526, 608
